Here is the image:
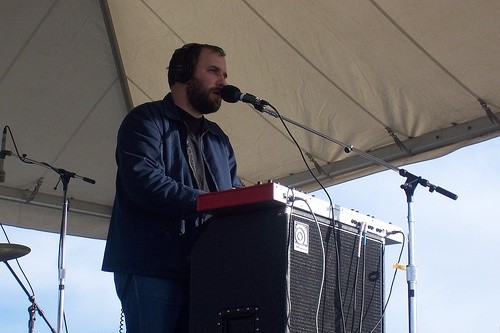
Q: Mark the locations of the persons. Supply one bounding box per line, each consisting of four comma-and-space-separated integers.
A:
101, 43, 244, 333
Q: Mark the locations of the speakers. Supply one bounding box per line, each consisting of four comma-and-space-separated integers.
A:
189, 206, 386, 333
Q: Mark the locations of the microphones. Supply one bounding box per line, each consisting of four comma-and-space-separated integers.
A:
0, 127, 7, 183
221, 85, 269, 105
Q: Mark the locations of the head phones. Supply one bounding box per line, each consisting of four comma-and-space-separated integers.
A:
170, 44, 193, 83
